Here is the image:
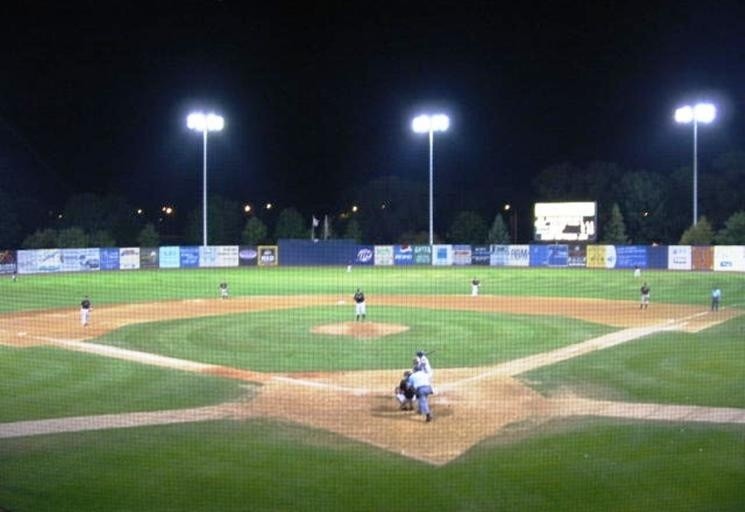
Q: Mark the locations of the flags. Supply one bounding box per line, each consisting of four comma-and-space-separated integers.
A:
313, 216, 320, 227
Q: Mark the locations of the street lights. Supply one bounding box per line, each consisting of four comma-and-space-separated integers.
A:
412, 111, 450, 245
184, 111, 227, 246
674, 102, 716, 225
504, 202, 518, 243
260, 203, 272, 219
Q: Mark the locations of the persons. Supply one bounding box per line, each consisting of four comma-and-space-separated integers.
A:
81, 296, 93, 327
352, 287, 366, 322
220, 279, 228, 300
346, 258, 352, 273
639, 282, 650, 310
711, 285, 721, 312
471, 276, 480, 296
634, 266, 640, 277
12, 269, 17, 283
395, 350, 435, 423
